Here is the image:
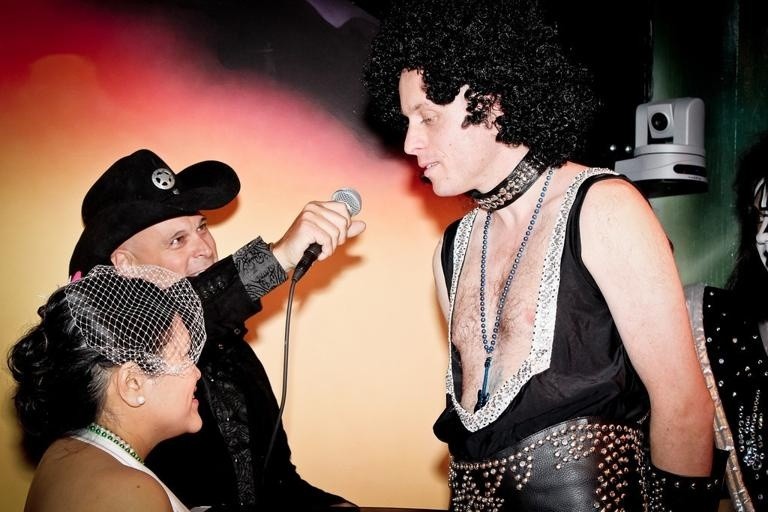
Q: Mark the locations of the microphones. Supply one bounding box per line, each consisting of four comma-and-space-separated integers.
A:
292, 187, 362, 279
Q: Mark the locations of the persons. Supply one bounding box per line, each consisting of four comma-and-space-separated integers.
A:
675, 172, 768, 511
361, 9, 716, 511
7, 276, 204, 512
68, 148, 366, 512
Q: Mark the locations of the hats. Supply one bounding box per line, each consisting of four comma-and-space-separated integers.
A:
80, 148, 240, 264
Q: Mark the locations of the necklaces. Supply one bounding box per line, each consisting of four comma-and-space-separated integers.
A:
471, 150, 548, 215
472, 169, 554, 413
81, 423, 143, 465
